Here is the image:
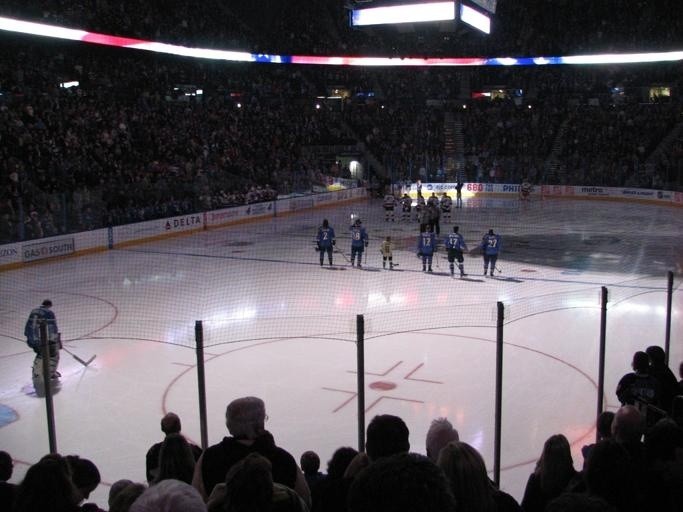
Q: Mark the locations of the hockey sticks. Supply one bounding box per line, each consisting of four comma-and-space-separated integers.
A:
455, 261, 467, 275
392, 263, 399, 265
62, 346, 97, 367
334, 243, 351, 262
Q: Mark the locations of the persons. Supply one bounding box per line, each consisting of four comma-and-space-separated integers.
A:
24, 300, 62, 396
